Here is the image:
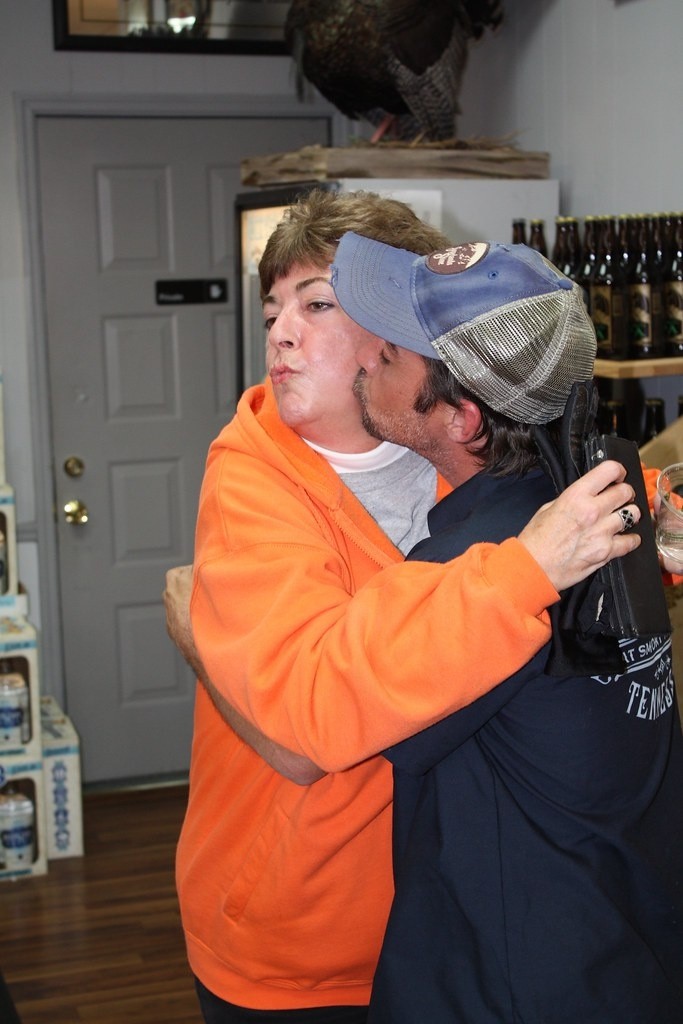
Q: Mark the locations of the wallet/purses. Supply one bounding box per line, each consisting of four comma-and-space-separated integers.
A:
580, 433, 674, 643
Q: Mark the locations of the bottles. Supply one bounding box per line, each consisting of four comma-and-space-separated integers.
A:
513, 203, 683, 448
2, 660, 30, 749
0, 780, 37, 877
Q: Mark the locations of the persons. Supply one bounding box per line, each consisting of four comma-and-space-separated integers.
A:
176, 183, 645, 1024
161, 228, 683, 1024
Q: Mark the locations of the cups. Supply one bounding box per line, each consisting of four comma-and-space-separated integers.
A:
656, 461, 683, 568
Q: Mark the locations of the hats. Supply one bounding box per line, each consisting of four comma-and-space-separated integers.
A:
332, 232, 597, 424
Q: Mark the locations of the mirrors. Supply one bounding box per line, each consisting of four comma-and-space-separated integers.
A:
50, 0, 295, 56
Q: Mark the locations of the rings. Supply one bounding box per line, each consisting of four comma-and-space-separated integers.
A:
615, 507, 636, 534
